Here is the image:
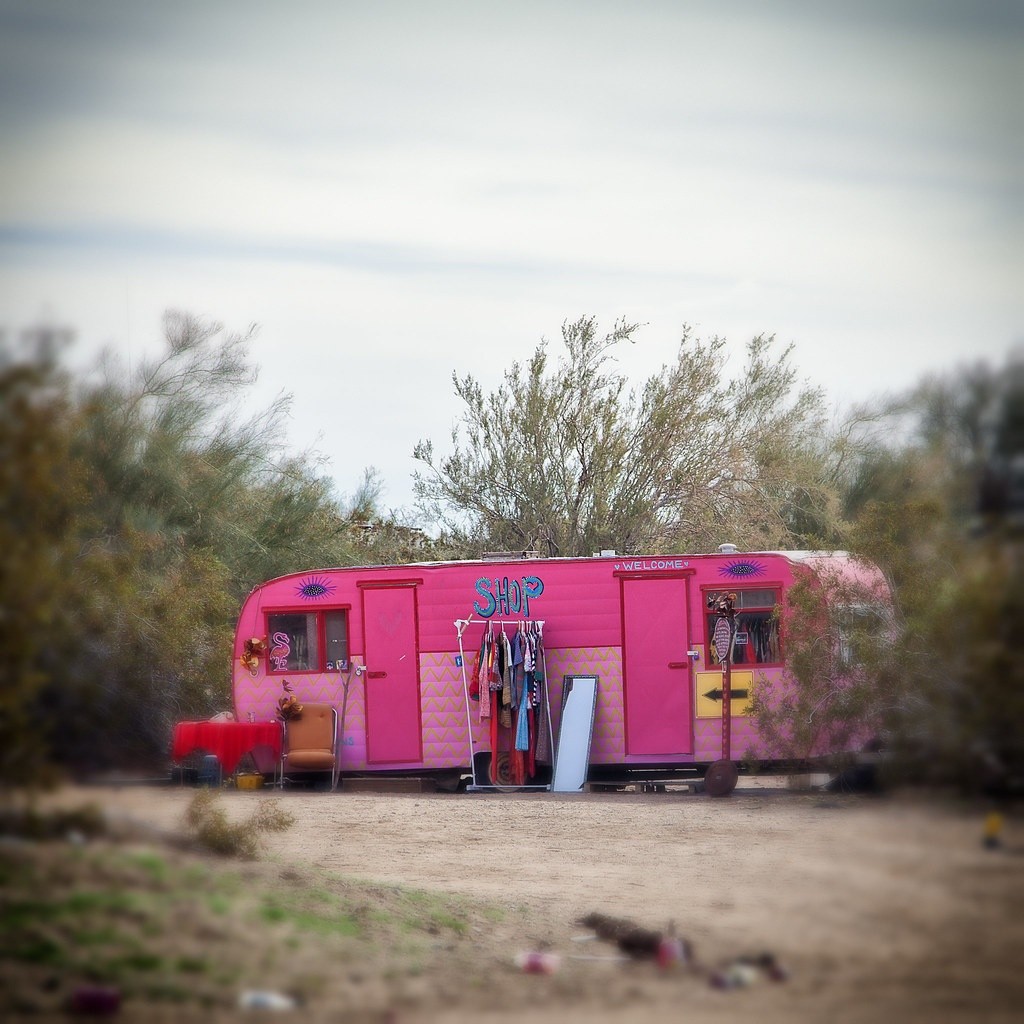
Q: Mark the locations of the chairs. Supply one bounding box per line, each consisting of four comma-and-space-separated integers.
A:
279, 703, 337, 790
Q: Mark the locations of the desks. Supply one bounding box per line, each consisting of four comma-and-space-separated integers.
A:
173, 721, 283, 791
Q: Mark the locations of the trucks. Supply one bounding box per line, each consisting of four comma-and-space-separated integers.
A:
232, 543, 896, 793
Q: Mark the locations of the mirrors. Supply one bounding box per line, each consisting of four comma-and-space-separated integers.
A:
549, 674, 600, 794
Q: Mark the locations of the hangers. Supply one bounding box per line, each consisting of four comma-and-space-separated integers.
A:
485, 620, 540, 642
742, 612, 777, 629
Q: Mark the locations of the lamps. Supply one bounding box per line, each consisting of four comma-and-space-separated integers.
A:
355, 668, 362, 676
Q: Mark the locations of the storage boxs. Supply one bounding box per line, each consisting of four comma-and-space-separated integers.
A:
236, 774, 264, 791
